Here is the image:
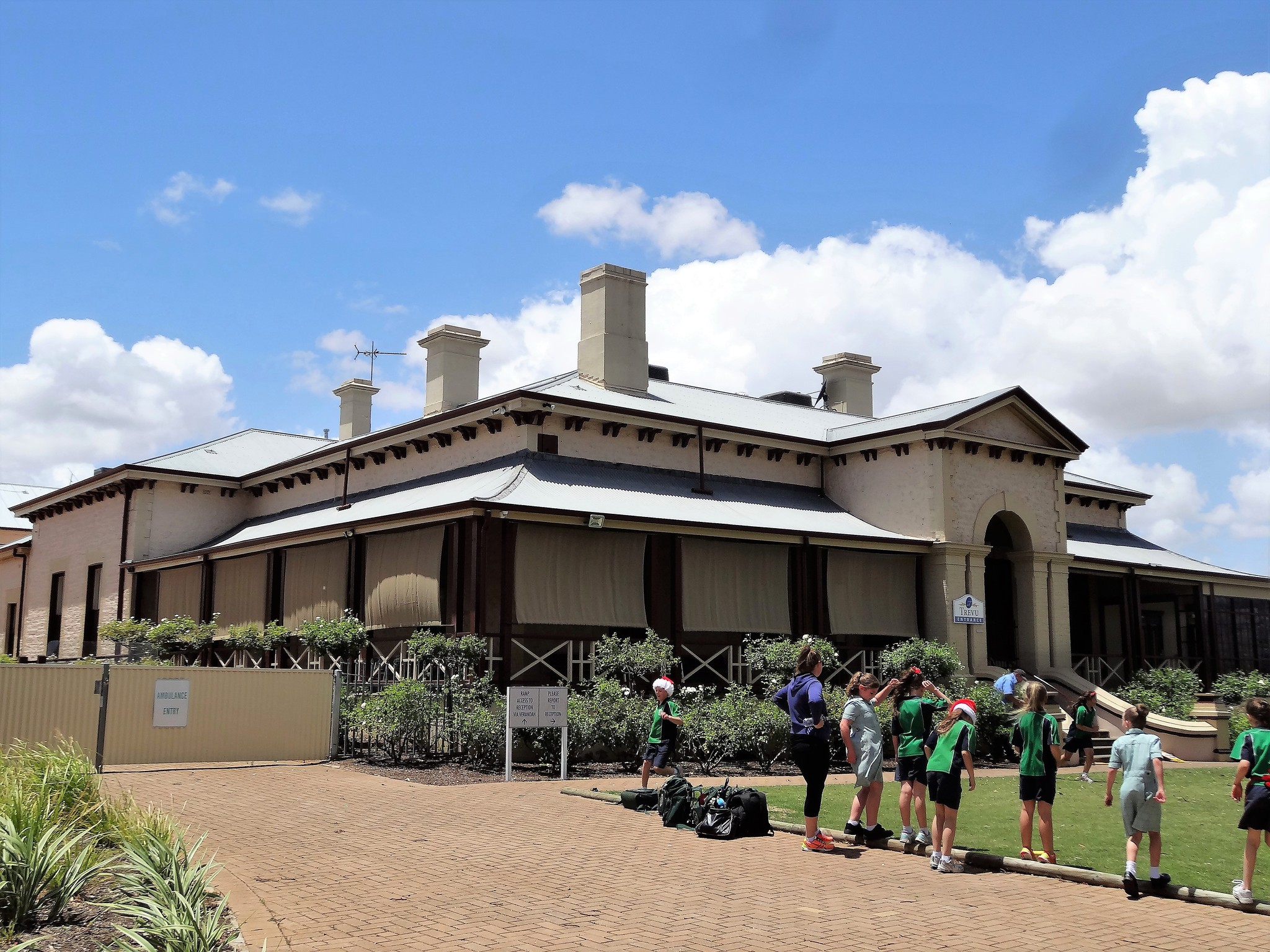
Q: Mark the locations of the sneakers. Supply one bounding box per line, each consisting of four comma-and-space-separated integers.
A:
915, 831, 933, 845
800, 837, 835, 853
1080, 775, 1094, 783
1020, 848, 1036, 861
930, 855, 941, 867
1038, 850, 1057, 864
815, 827, 833, 843
937, 859, 964, 873
900, 829, 916, 844
863, 823, 894, 840
1231, 879, 1254, 904
844, 818, 865, 835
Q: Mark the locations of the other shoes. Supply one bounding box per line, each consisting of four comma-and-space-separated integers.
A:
1123, 872, 1139, 896
676, 766, 683, 777
1150, 873, 1171, 887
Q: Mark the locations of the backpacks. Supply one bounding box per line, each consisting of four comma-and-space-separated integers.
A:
727, 787, 774, 839
691, 778, 733, 826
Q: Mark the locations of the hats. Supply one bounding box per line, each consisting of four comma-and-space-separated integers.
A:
950, 699, 976, 725
652, 676, 674, 697
1014, 669, 1028, 681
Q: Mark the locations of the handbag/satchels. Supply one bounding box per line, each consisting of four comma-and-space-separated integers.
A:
657, 776, 694, 827
694, 786, 733, 837
621, 788, 658, 811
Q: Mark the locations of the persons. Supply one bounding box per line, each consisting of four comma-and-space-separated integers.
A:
1229, 697, 1270, 904
641, 676, 683, 788
924, 699, 976, 873
891, 666, 953, 845
771, 645, 834, 852
994, 669, 1027, 763
1104, 703, 1171, 899
1061, 690, 1099, 782
1009, 682, 1061, 864
839, 671, 903, 839
